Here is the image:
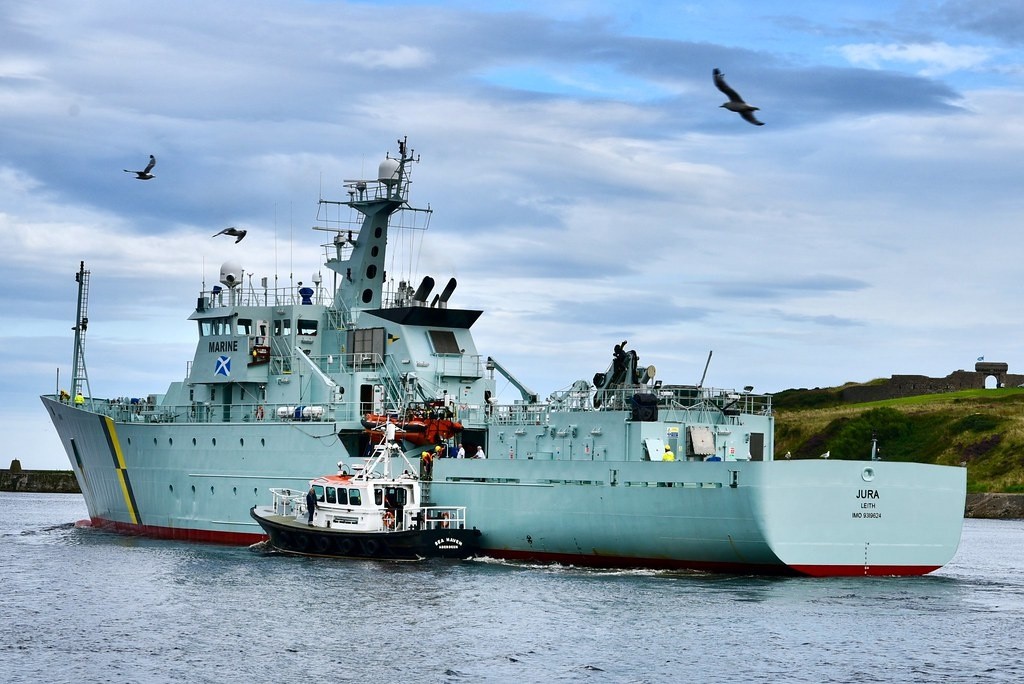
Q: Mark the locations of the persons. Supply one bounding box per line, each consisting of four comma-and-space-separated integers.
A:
307, 488, 318, 527
60, 389, 70, 404
378, 492, 392, 507
473, 446, 486, 482
662, 445, 675, 487
75, 393, 84, 407
106, 397, 120, 409
138, 398, 145, 405
422, 452, 432, 472
435, 446, 445, 456
457, 444, 465, 459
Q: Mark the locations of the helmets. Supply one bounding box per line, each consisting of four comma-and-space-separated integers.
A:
665, 445, 671, 450
435, 445, 441, 452
422, 452, 428, 457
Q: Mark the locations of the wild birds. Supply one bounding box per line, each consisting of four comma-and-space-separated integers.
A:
124, 155, 157, 180
212, 227, 247, 244
713, 68, 765, 126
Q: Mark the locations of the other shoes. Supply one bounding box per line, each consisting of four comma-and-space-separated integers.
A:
308, 524, 314, 527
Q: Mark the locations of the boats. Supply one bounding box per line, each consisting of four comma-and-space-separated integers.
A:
41, 137, 969, 574
250, 415, 482, 561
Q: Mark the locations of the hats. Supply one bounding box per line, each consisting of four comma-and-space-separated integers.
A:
477, 446, 482, 449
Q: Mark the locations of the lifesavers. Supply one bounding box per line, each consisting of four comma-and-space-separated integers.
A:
442, 513, 450, 526
383, 512, 396, 526
255, 405, 264, 419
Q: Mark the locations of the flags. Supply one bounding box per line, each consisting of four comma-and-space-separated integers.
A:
978, 357, 984, 361
